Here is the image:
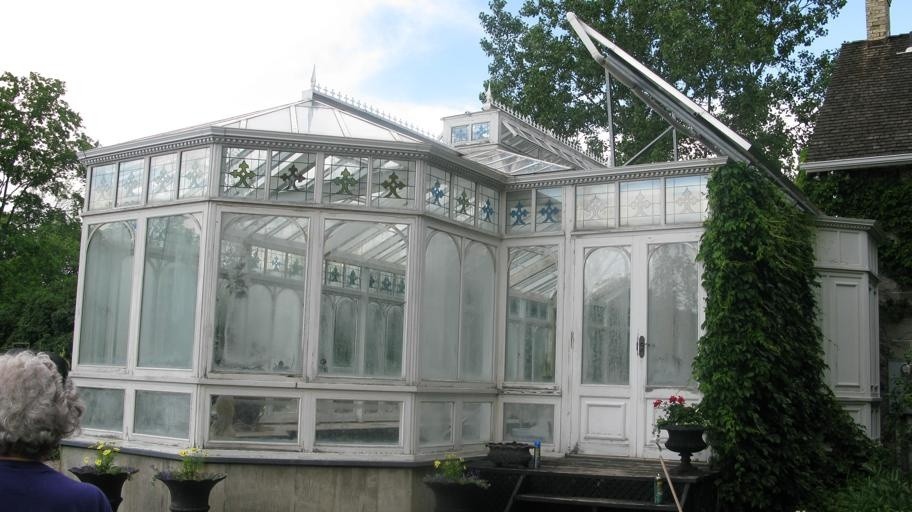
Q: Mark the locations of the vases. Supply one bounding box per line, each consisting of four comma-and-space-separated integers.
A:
483, 439, 535, 467
422, 477, 488, 512
82, 438, 123, 473
67, 463, 141, 512
659, 424, 707, 474
154, 470, 232, 511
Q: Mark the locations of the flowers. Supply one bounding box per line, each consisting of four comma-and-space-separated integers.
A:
431, 451, 480, 480
652, 393, 706, 426
151, 447, 210, 479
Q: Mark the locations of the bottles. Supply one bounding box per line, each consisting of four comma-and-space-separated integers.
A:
533, 440, 541, 470
654, 473, 663, 505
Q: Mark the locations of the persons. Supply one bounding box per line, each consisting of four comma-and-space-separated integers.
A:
0, 351, 115, 511
